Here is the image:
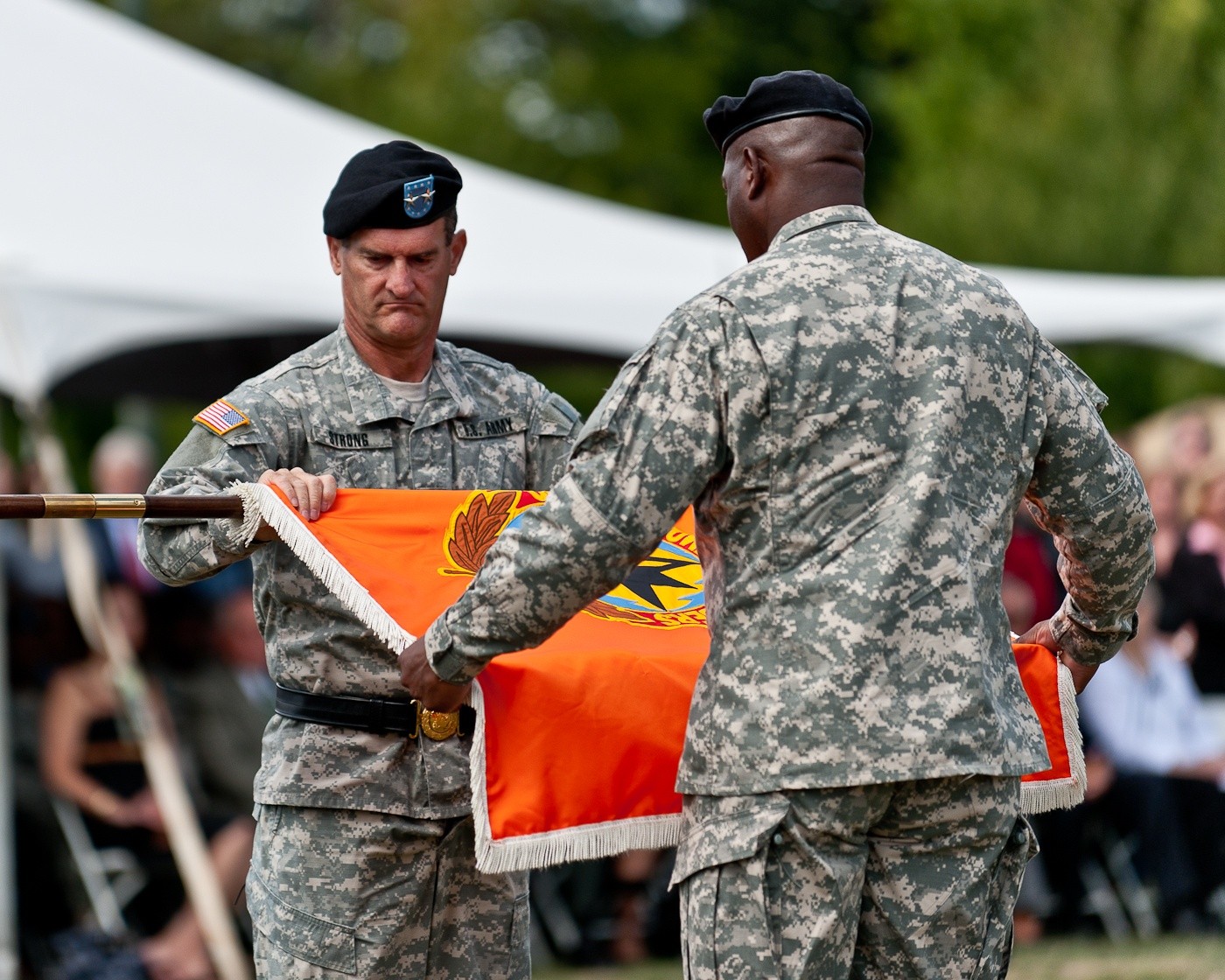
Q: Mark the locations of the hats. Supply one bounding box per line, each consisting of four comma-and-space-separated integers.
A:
323, 141, 462, 240
704, 70, 873, 157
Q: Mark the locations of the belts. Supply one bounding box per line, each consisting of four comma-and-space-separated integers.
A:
273, 682, 477, 741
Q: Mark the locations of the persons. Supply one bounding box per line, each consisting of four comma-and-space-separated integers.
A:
140, 135, 586, 980
396, 72, 1158, 980
0, 391, 1225, 980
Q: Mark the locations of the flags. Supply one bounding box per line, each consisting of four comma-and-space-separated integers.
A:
209, 474, 1087, 874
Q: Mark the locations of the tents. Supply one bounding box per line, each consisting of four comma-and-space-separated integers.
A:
0, 0, 1225, 980
